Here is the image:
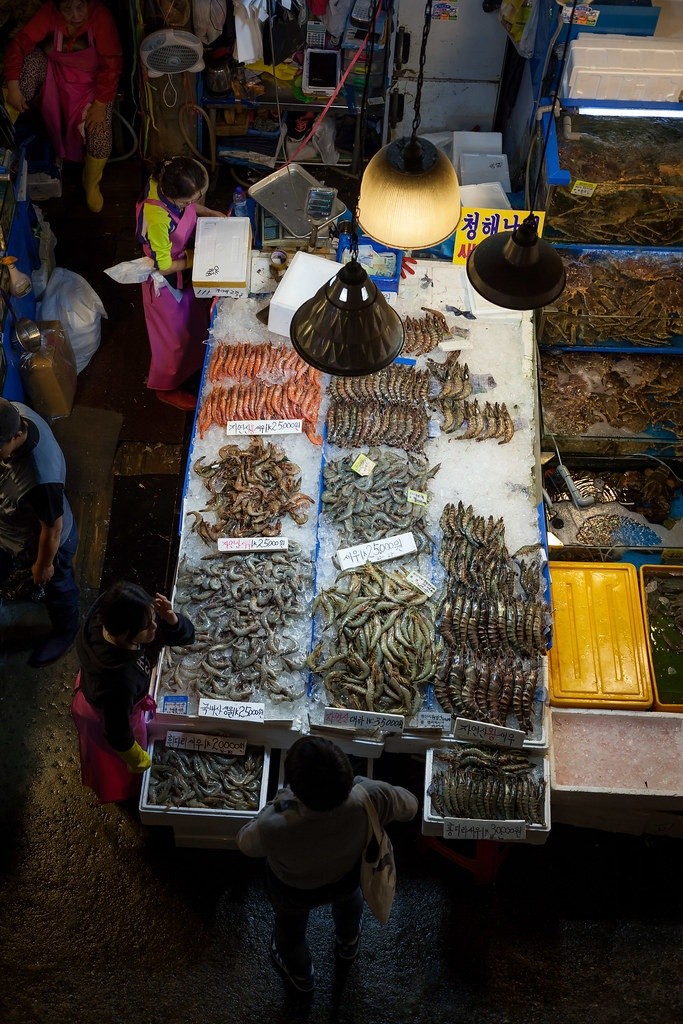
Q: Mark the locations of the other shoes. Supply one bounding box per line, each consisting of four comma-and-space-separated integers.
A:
29, 627, 80, 667
271, 933, 314, 992
155, 385, 198, 411
338, 936, 358, 960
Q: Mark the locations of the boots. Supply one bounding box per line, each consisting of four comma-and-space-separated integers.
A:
81, 153, 108, 213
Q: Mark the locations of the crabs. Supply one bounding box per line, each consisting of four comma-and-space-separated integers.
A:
539, 141, 683, 457
645, 576, 683, 653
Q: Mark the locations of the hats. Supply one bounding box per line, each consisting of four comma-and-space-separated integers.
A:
0, 397, 19, 441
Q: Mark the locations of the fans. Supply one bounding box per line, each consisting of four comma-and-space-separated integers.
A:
139, 28, 206, 78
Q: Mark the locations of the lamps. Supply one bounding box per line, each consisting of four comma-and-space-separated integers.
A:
358, 0, 461, 253
463, 0, 577, 310
290, 0, 409, 381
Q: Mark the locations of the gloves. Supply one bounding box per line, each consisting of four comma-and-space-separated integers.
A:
115, 741, 151, 773
185, 249, 193, 268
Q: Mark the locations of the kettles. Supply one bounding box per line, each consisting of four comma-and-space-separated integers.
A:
207, 56, 232, 99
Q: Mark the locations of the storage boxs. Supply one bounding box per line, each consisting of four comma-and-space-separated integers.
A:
337, 232, 403, 295
543, 455, 683, 566
31, 260, 49, 301
0, 181, 16, 252
341, 71, 383, 88
546, 562, 654, 712
343, 49, 385, 63
548, 706, 683, 813
216, 114, 250, 136
218, 110, 289, 172
382, 656, 548, 758
421, 746, 552, 846
551, 804, 653, 836
247, 162, 347, 241
540, 97, 683, 247
539, 243, 683, 354
401, 731, 454, 740
300, 729, 386, 759
537, 354, 683, 461
28, 173, 62, 198
344, 59, 384, 75
36, 302, 42, 321
639, 563, 683, 713
267, 250, 346, 338
137, 733, 271, 826
173, 826, 241, 849
548, 4, 662, 78
523, 721, 547, 746
277, 749, 373, 791
302, 48, 341, 100
143, 666, 300, 750
644, 811, 683, 839
340, 11, 387, 52
558, 40, 683, 103
192, 216, 253, 299
17, 319, 79, 420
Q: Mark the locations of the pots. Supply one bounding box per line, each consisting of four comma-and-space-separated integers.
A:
0, 288, 41, 353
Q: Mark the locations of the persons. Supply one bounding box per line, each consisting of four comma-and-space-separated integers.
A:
136, 155, 228, 411
71, 583, 195, 803
6, 0, 121, 213
237, 737, 419, 991
0, 397, 80, 667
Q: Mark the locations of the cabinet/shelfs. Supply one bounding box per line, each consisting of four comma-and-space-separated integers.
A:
197, 72, 384, 207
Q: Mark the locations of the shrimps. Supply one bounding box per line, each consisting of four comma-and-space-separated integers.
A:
425, 318, 439, 353
416, 319, 431, 357
308, 560, 443, 718
406, 317, 424, 354
435, 501, 547, 735
421, 307, 450, 346
199, 341, 323, 445
161, 436, 315, 704
321, 446, 440, 566
400, 315, 416, 355
426, 350, 515, 445
431, 748, 546, 826
148, 747, 263, 810
326, 363, 430, 454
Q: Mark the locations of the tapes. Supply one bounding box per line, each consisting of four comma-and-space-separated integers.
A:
270, 249, 289, 270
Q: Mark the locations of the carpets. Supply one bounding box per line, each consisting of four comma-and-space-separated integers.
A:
75, 170, 236, 445
97, 472, 181, 601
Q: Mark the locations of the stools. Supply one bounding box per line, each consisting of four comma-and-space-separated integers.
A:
418, 836, 502, 880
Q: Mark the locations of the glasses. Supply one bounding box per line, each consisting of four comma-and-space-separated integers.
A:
173, 191, 203, 209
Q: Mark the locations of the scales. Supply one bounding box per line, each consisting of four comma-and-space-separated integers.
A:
249, 161, 348, 246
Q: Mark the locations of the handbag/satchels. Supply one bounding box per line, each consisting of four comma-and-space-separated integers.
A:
353, 784, 395, 924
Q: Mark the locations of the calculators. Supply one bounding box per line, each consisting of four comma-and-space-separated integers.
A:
353, 0, 373, 21
306, 21, 327, 50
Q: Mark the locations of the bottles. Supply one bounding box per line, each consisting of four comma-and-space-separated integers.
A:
233, 187, 247, 218
